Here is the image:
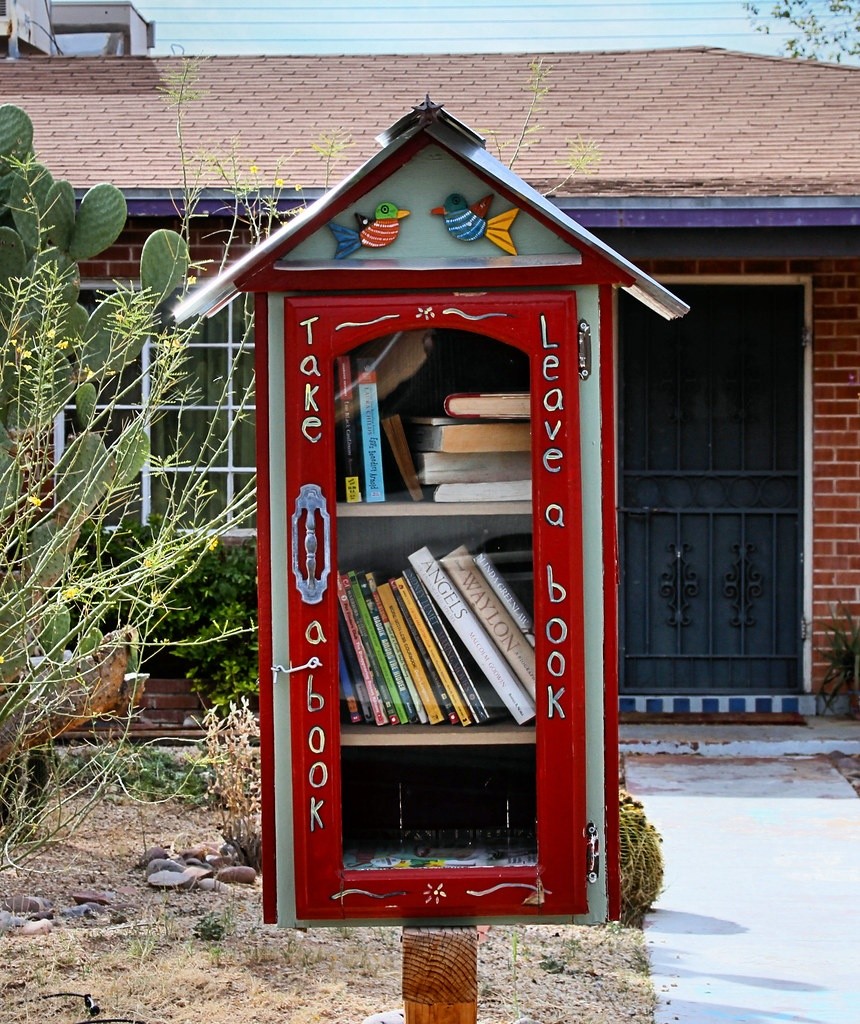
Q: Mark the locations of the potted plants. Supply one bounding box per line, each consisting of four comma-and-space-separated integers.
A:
812, 598, 860, 719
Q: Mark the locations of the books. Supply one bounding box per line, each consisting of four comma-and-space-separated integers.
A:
337, 545, 536, 725
337, 352, 532, 503
